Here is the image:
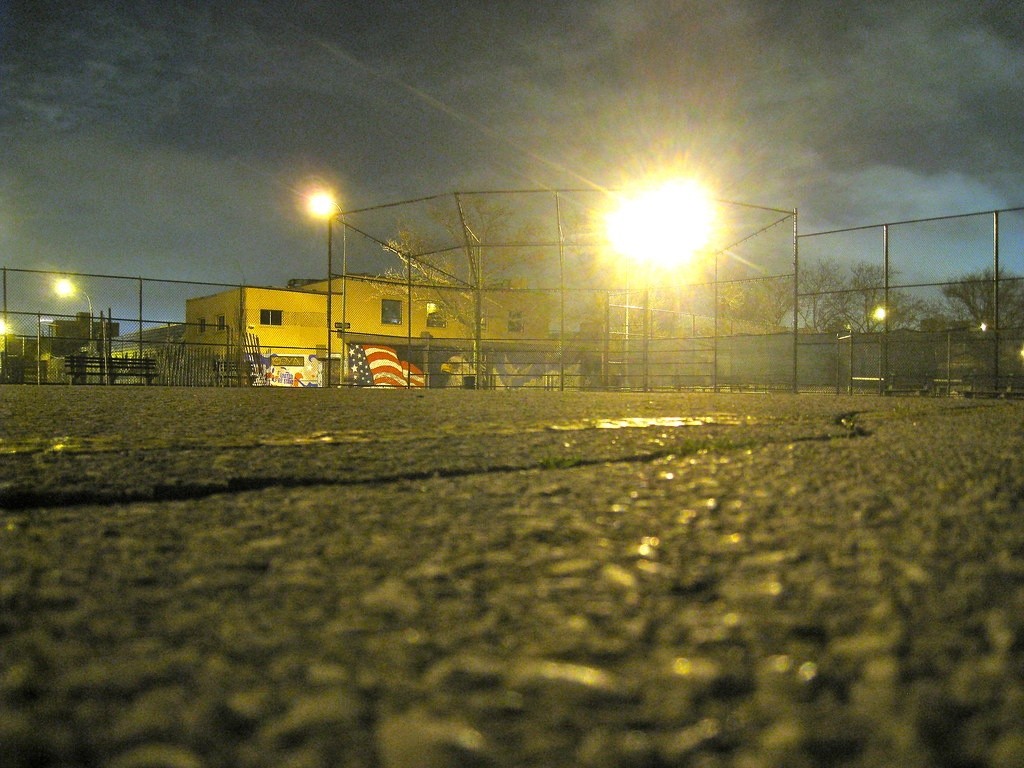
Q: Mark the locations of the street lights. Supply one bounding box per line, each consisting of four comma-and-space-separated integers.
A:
54, 280, 93, 384
310, 193, 346, 387
874, 308, 886, 396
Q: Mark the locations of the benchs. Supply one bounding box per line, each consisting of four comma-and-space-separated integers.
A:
65, 355, 160, 386
213, 360, 258, 386
882, 374, 1024, 400
